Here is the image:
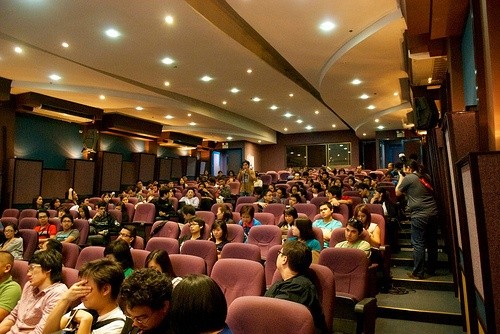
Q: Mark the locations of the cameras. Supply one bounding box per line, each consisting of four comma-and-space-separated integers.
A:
390, 153, 407, 178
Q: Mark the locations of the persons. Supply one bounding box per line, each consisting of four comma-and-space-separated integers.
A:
82, 148, 92, 160
42, 258, 126, 334
237, 160, 257, 196
0, 187, 115, 266
257, 153, 430, 258
395, 159, 438, 279
0, 249, 69, 334
263, 240, 316, 307
171, 275, 233, 334
117, 268, 173, 334
239, 205, 262, 243
103, 170, 236, 288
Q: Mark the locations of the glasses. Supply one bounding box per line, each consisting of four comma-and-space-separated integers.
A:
63, 309, 79, 332
124, 314, 148, 323
26, 265, 41, 274
119, 233, 130, 237
350, 218, 361, 223
39, 216, 48, 218
277, 249, 287, 256
189, 223, 199, 226
61, 220, 72, 223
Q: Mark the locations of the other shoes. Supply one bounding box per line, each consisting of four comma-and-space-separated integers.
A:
410, 274, 422, 280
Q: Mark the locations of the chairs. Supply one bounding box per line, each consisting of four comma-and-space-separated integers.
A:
0, 166, 400, 334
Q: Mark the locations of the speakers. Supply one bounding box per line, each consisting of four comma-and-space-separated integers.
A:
412, 96, 439, 130
